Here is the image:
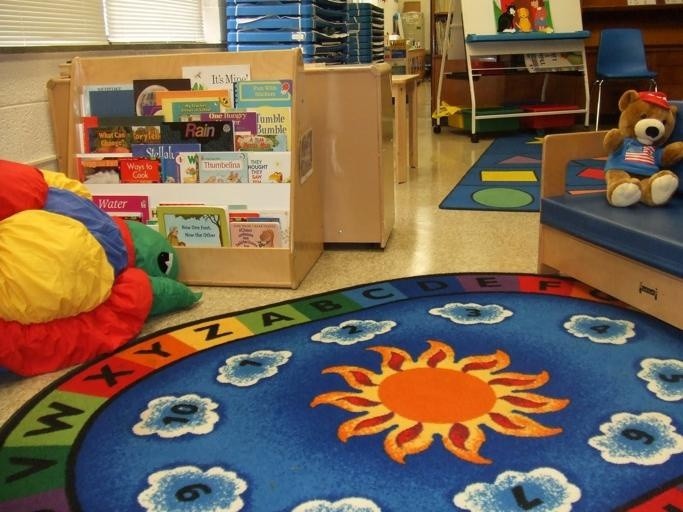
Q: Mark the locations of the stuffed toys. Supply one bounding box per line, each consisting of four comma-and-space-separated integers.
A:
603, 89, 683, 208
0, 159, 203, 377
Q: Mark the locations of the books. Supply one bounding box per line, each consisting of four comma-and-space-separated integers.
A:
79, 78, 290, 248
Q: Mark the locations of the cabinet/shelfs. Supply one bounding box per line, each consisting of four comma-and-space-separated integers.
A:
62, 46, 325, 292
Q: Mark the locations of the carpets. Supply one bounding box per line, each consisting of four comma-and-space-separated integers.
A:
2, 267, 683, 512
437, 133, 543, 212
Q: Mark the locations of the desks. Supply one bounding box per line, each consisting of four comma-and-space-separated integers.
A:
389, 73, 423, 186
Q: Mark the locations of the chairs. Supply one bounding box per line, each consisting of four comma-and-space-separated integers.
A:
593, 28, 659, 130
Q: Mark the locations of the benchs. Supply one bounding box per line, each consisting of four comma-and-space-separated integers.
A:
536, 102, 682, 334
